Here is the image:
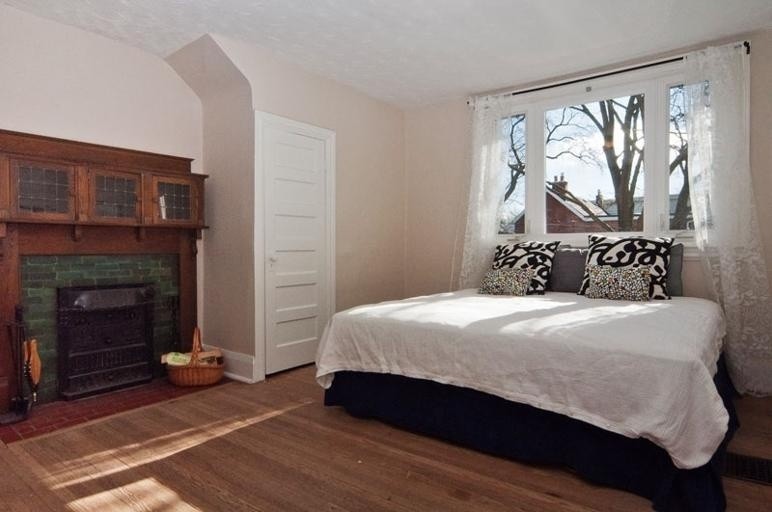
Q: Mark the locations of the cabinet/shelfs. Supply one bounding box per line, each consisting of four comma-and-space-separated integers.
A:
0, 129, 209, 243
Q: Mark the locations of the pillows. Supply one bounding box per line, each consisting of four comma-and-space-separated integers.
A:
491, 239, 561, 294
588, 263, 650, 300
576, 235, 673, 300
479, 268, 533, 295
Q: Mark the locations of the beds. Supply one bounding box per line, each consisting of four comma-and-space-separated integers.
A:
313, 234, 739, 510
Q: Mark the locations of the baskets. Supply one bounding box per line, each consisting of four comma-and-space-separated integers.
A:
164, 327, 225, 388
